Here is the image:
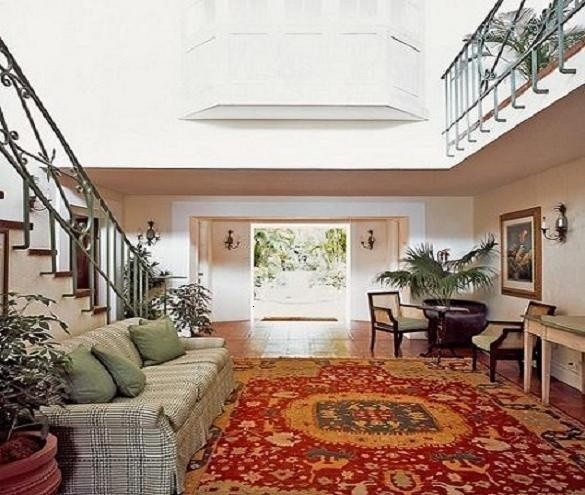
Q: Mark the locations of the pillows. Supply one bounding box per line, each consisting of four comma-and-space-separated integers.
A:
129, 315, 186, 366
54, 344, 146, 404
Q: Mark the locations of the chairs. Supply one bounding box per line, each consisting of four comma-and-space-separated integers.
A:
368, 291, 556, 383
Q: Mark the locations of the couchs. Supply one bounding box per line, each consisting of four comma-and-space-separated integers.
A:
34, 316, 234, 495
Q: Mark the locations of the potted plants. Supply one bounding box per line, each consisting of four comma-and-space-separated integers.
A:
0, 292, 73, 495
376, 233, 503, 346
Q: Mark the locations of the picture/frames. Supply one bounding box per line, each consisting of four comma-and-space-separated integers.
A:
499, 207, 541, 301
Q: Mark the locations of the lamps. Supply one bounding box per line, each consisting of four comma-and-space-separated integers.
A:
28, 195, 52, 212
138, 220, 160, 246
224, 230, 240, 250
361, 230, 376, 250
540, 202, 568, 243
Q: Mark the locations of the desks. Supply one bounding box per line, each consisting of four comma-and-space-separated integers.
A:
520, 314, 585, 405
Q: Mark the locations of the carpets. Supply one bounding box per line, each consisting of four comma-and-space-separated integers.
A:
181, 356, 585, 495
